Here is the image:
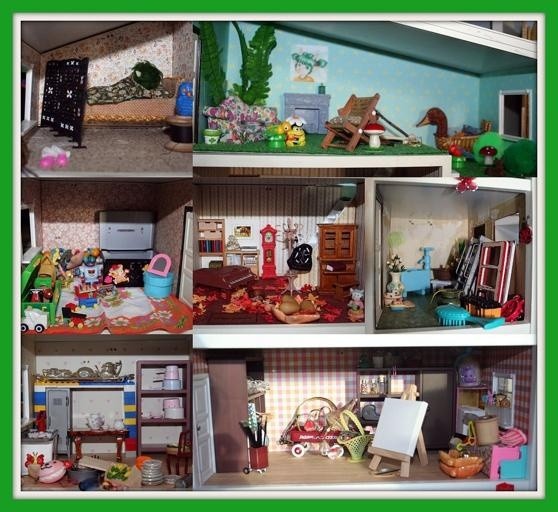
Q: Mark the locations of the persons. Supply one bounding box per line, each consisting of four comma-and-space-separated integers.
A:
347, 288, 365, 323
21, 244, 127, 334
